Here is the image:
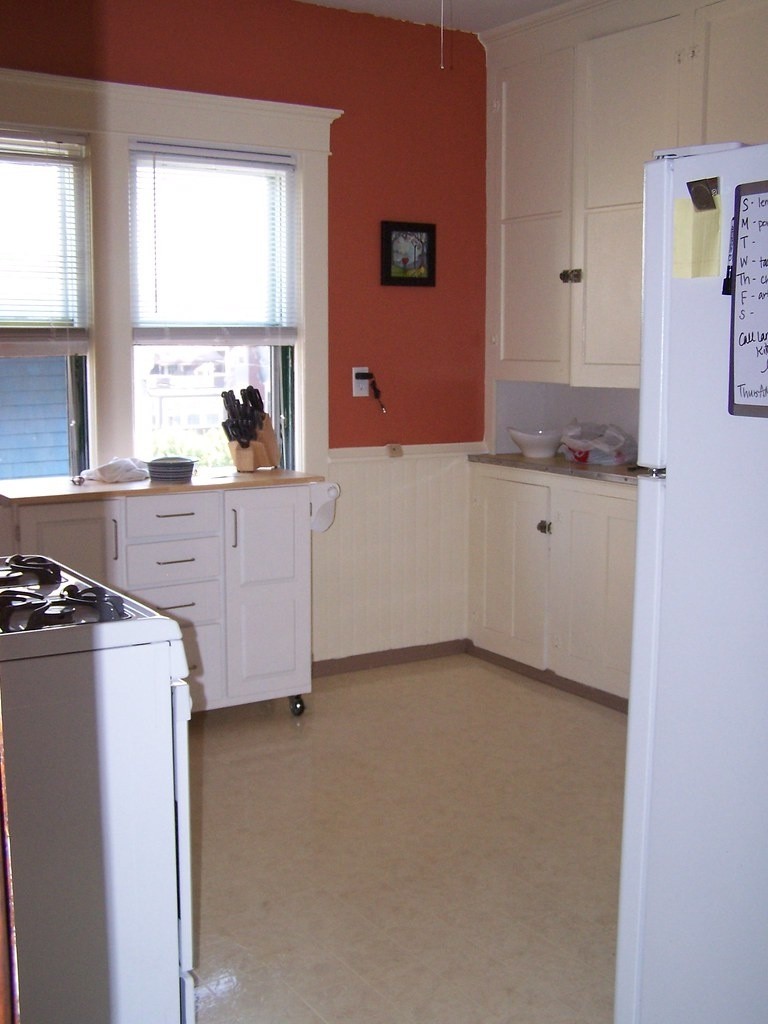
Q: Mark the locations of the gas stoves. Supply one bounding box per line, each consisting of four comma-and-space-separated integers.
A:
0, 554, 183, 636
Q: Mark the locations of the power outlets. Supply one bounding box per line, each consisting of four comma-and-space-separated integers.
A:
352, 367, 369, 397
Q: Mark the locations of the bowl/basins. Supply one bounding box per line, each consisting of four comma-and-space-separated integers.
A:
141, 457, 199, 484
505, 426, 565, 458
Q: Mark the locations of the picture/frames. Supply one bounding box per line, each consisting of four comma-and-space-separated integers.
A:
380, 220, 436, 287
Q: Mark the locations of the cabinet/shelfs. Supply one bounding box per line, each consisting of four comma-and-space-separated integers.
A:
0, 499, 123, 594
224, 485, 311, 699
468, 461, 637, 700
486, 14, 682, 389
690, 0, 768, 146
125, 491, 222, 704
